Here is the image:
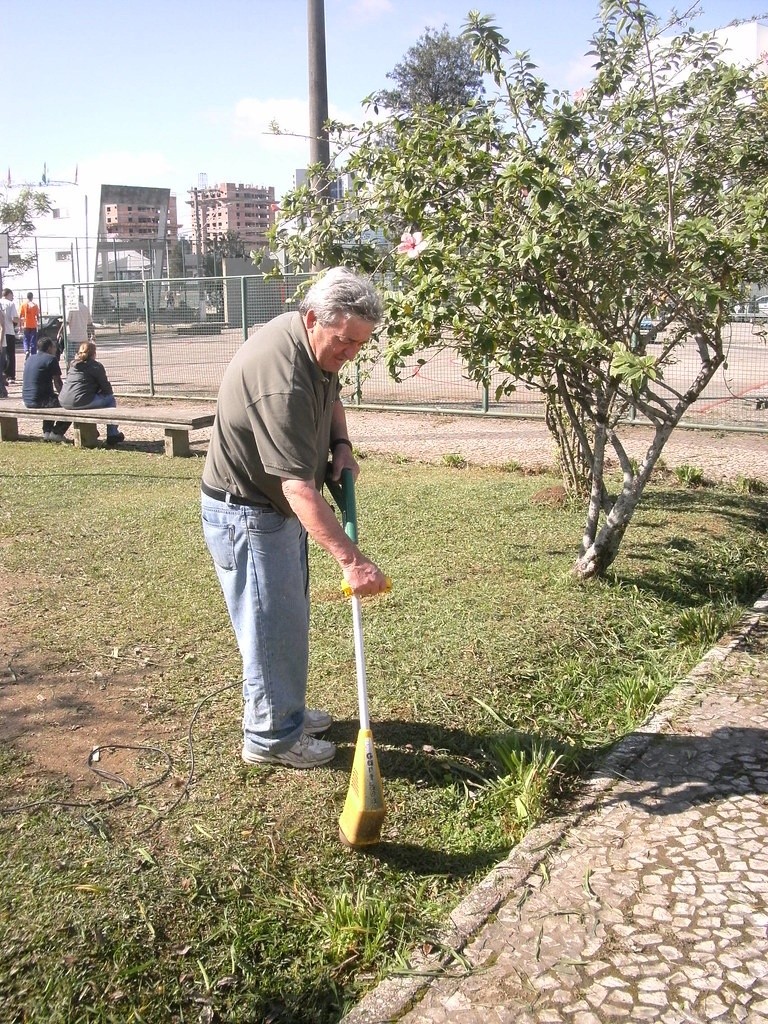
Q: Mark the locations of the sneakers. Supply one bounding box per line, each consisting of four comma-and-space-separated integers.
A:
96, 430, 100, 438
3, 372, 16, 384
43, 431, 66, 442
301, 704, 333, 734
241, 729, 336, 768
107, 431, 125, 446
0, 390, 8, 399
25, 351, 30, 361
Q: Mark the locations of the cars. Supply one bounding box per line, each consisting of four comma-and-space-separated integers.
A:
733, 296, 768, 314
639, 322, 657, 344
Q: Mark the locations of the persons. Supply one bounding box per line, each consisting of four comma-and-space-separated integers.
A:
0, 304, 8, 401
0, 288, 20, 384
18, 291, 39, 360
198, 265, 393, 769
56, 301, 93, 376
57, 342, 125, 447
22, 338, 74, 443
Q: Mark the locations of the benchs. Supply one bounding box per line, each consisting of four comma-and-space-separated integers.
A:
0, 396, 216, 458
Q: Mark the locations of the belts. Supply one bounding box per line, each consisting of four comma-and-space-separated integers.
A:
201, 480, 272, 509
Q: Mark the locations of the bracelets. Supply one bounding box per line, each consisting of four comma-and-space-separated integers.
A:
329, 438, 354, 455
